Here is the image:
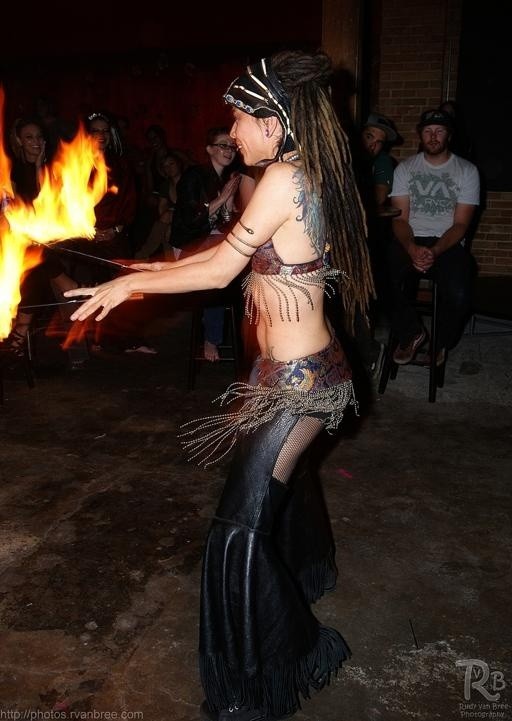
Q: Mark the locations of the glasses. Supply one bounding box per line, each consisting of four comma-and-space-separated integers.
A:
206, 142, 239, 153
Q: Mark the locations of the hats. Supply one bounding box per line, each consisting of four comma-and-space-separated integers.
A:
360, 112, 397, 144
417, 110, 454, 129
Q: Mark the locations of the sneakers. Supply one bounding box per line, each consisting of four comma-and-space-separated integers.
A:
360, 340, 387, 381
391, 324, 427, 366
422, 343, 445, 368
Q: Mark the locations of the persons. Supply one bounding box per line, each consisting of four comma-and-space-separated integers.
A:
10, 106, 480, 365
64, 49, 377, 721
